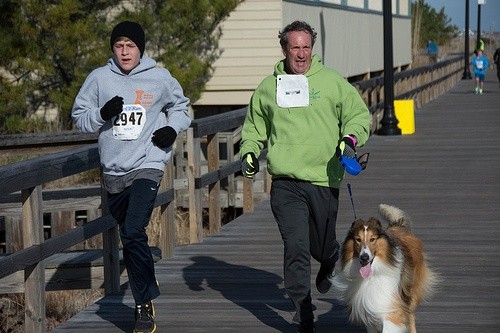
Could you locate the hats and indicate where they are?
[110,21,145,59]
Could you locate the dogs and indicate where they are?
[327,202,445,333]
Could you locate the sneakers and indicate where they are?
[316,265,335,294]
[133,300,156,333]
[298,322,316,333]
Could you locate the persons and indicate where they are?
[472,39,488,95]
[240,21,369,333]
[71,20,192,333]
[493,48,500,87]
[428,38,438,65]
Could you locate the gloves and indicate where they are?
[152,126,177,148]
[241,152,255,178]
[338,137,356,159]
[100,96,124,121]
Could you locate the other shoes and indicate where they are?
[479,89,482,93]
[475,87,479,92]
